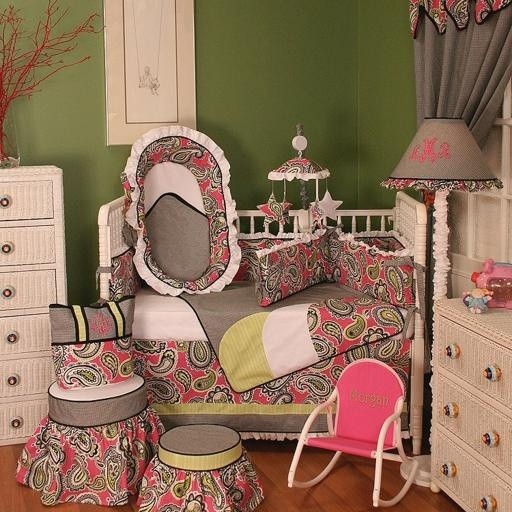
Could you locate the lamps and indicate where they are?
[379,116,504,488]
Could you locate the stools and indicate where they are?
[48,376,242,511]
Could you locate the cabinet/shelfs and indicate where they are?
[431,299,512,512]
[0,164,70,447]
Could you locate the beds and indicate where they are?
[95,189,432,455]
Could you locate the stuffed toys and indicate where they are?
[464,287,496,314]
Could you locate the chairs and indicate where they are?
[286,359,420,509]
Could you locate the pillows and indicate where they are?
[48,296,137,391]
[252,225,417,311]
